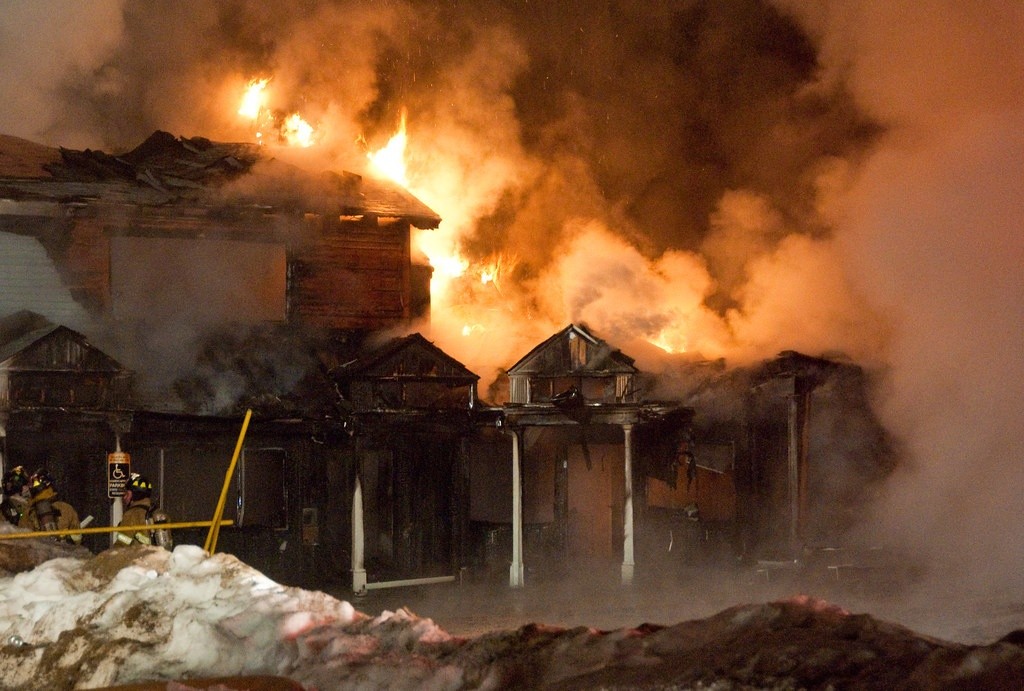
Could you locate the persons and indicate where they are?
[1,466,83,545]
[112,475,173,548]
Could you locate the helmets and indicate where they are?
[125,476,153,507]
[4,465,53,500]
